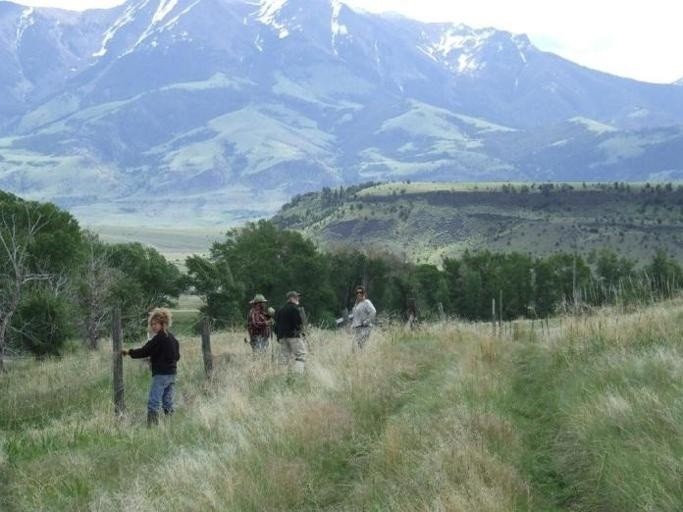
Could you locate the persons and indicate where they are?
[349,284,377,356]
[121,306,181,429]
[245,293,275,362]
[274,289,310,379]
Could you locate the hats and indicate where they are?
[248,292,269,305]
[286,291,302,298]
